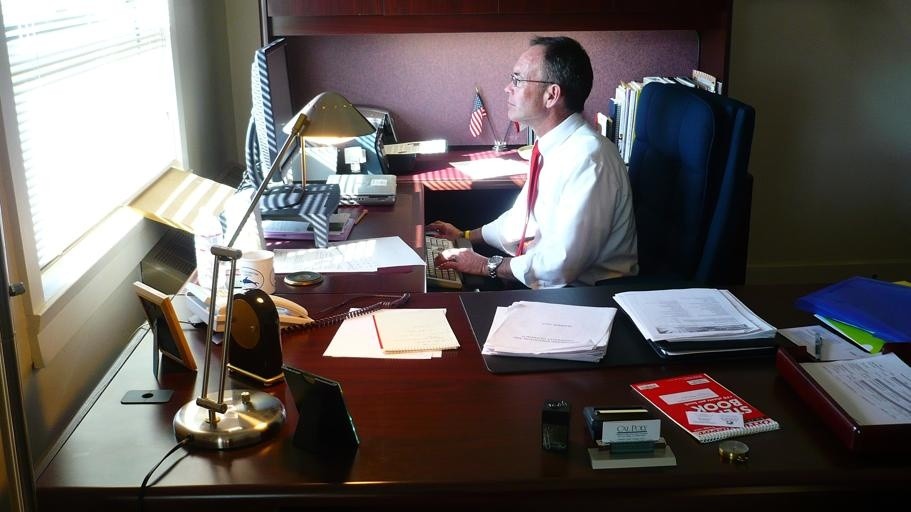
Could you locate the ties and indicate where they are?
[515,140,542,256]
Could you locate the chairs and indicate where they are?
[600,81,757,286]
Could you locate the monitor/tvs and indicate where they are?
[246,37,304,208]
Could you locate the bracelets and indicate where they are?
[463,229,470,242]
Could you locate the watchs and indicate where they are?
[487,253,504,279]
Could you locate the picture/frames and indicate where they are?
[278,360,363,484]
[131,280,198,371]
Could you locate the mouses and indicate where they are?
[425,229,440,236]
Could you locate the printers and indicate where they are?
[293,104,419,182]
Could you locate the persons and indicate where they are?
[425,35,641,291]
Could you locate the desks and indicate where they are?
[31,142,911,509]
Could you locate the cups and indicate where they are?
[237,249,277,297]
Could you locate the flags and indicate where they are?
[466,93,488,140]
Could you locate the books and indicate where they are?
[595,68,722,168]
[630,370,781,446]
[325,174,397,208]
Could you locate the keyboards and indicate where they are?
[425,236,464,289]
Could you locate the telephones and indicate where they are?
[185,282,315,333]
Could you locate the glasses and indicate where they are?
[509,74,557,87]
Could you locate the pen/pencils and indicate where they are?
[813,333,823,359]
[354,209,369,226]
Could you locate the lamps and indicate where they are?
[172,91,377,452]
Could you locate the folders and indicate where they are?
[794,275,911,345]
